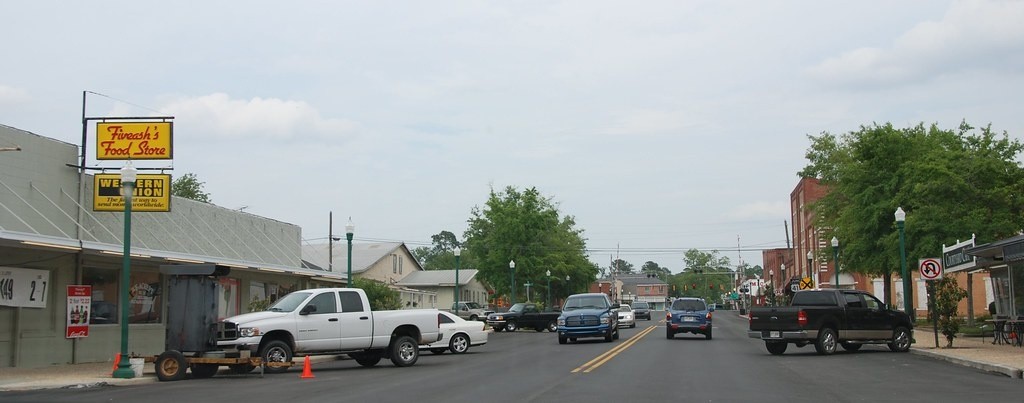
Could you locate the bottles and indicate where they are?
[71,304,88,325]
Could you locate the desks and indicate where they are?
[1006,319,1024,348]
[985,318,1011,347]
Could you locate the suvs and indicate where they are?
[557,292,621,344]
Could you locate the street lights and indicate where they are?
[344,214,354,288]
[743,281,747,315]
[566,275,571,296]
[509,259,516,305]
[629,291,631,306]
[806,250,813,289]
[546,269,551,312]
[831,236,840,289]
[894,207,916,344]
[454,246,460,316]
[769,269,774,307]
[112,157,136,379]
[621,289,624,303]
[748,278,752,308]
[781,263,786,307]
[599,283,602,292]
[757,274,760,306]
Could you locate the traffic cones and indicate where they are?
[111,353,122,374]
[298,355,316,379]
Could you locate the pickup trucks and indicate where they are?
[215,286,445,375]
[746,287,914,357]
[486,301,562,333]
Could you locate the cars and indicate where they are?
[665,296,715,340]
[632,301,653,321]
[449,301,495,324]
[418,309,489,355]
[617,303,636,328]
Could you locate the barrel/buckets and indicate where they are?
[129,358,145,377]
